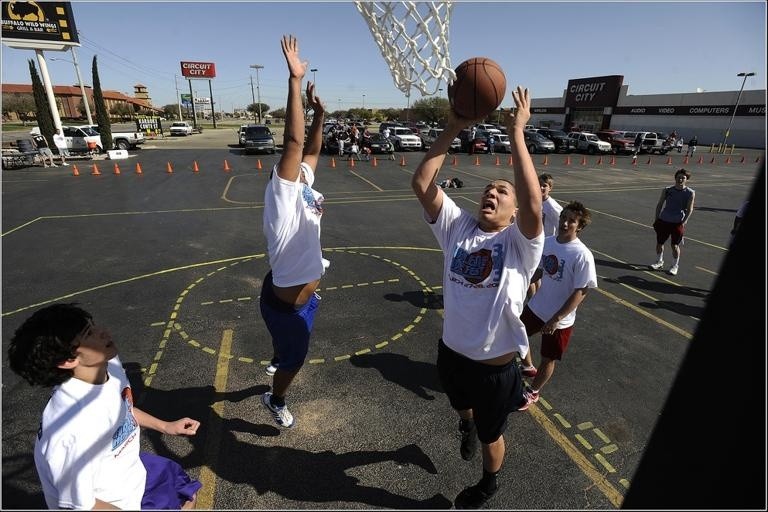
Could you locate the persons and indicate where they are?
[515,201,599,412]
[32,129,57,168]
[383,126,391,138]
[527,174,565,303]
[9,302,201,511]
[467,127,476,155]
[488,132,495,156]
[649,169,695,276]
[386,139,396,160]
[731,198,750,235]
[411,85,546,509]
[687,136,697,157]
[634,134,642,156]
[53,129,70,166]
[332,124,374,160]
[668,130,684,154]
[260,32,325,428]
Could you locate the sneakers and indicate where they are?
[458,419,477,462]
[517,386,539,411]
[521,365,538,377]
[454,479,501,510]
[647,261,665,270]
[260,392,295,429]
[264,362,278,376]
[668,264,680,275]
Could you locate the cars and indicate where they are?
[167,118,197,135]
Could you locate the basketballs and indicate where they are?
[448,57,506,120]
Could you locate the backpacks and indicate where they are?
[451,178,463,188]
[440,177,451,188]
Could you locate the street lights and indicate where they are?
[310,68,318,97]
[437,88,444,119]
[718,71,756,153]
[362,95,366,109]
[249,64,264,124]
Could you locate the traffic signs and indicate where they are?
[49,57,93,126]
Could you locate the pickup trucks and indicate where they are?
[30,125,147,151]
